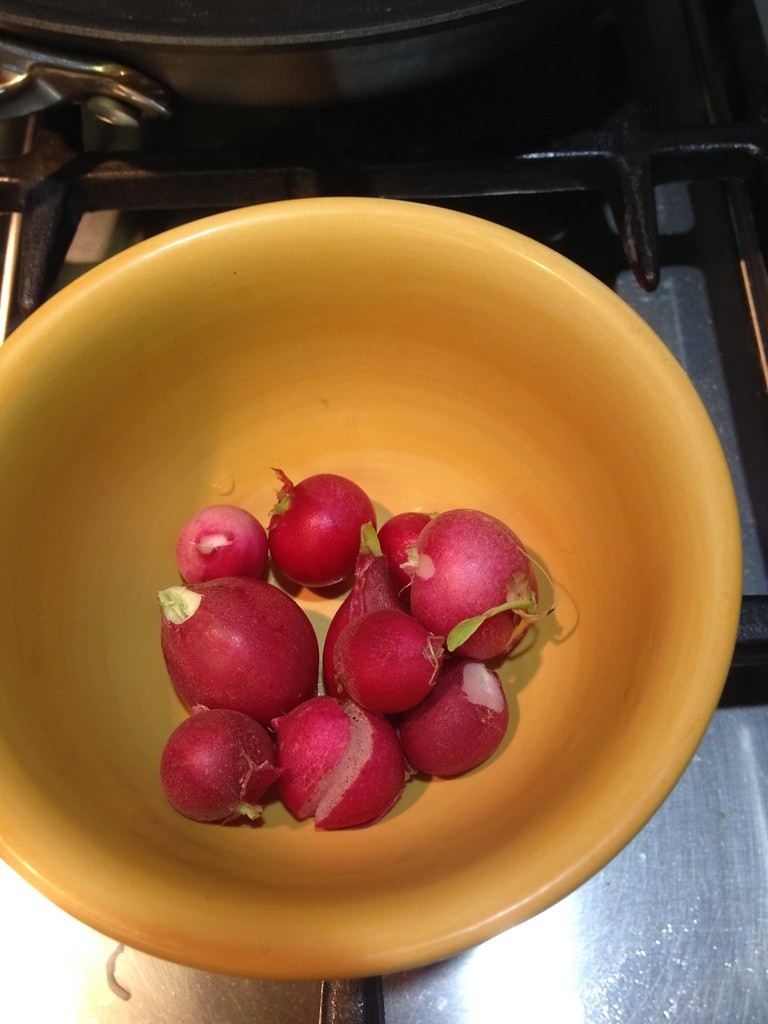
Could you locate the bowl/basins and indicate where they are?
[0,191,742,980]
